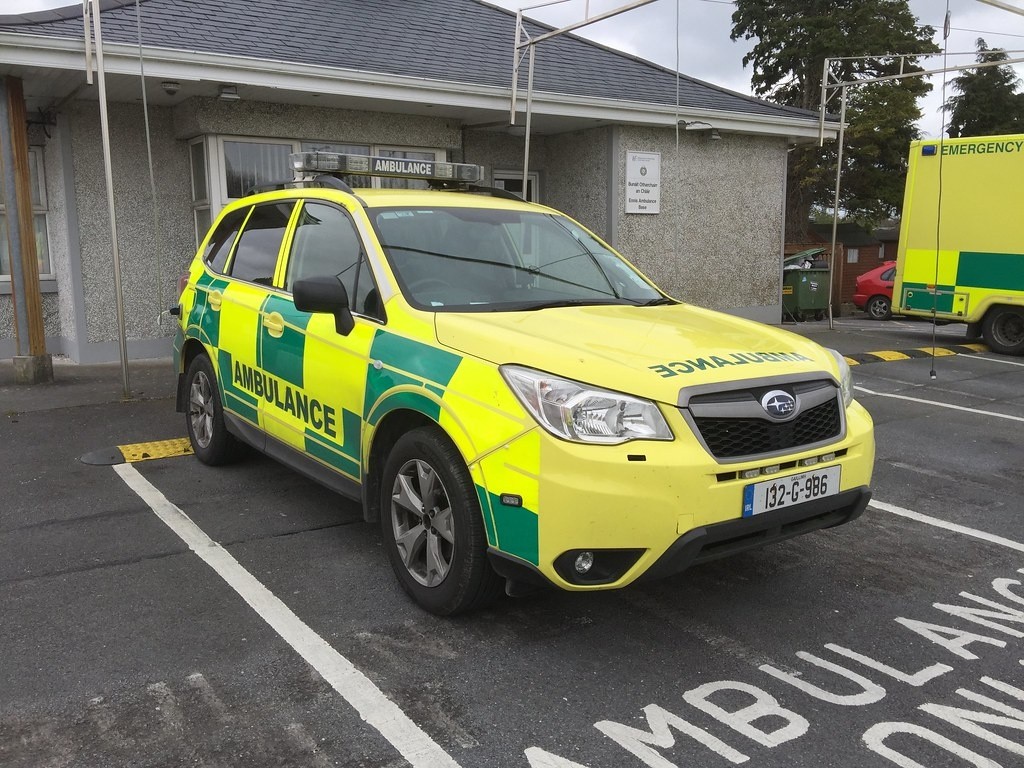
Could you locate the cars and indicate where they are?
[852,260,897,323]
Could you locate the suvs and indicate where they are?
[169,152,878,621]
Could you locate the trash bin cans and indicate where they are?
[782,248,829,321]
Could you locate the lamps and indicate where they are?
[217,85,241,105]
[708,129,721,139]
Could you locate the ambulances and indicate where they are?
[891,131,1024,355]
